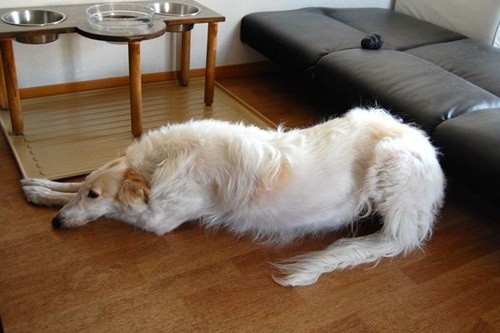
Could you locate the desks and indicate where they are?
[1,0,225,140]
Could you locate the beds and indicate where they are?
[243,0,499,213]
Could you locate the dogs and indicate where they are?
[19,105,448,289]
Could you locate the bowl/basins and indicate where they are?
[85,2,156,46]
[149,2,201,32]
[1,9,67,45]
